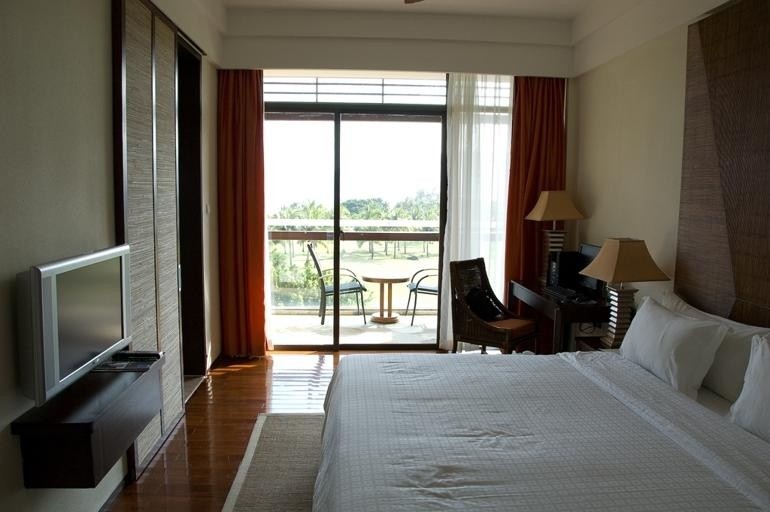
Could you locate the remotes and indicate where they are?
[112,351,163,359]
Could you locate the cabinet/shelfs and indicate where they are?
[9,350,166,487]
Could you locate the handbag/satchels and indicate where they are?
[465,286,505,320]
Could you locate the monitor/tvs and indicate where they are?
[574,242,603,297]
[16,243,134,408]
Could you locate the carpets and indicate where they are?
[221,410,325,511]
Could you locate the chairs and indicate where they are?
[307,241,367,325]
[450,258,541,354]
[403,269,440,326]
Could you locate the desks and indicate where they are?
[506,277,612,353]
[362,275,413,324]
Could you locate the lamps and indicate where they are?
[523,190,584,287]
[579,237,671,349]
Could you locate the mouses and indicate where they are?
[576,296,584,302]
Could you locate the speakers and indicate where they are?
[549,251,577,287]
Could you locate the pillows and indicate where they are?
[617,290,769,445]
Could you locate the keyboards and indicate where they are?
[543,287,577,301]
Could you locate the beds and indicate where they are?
[310,351,770,510]
